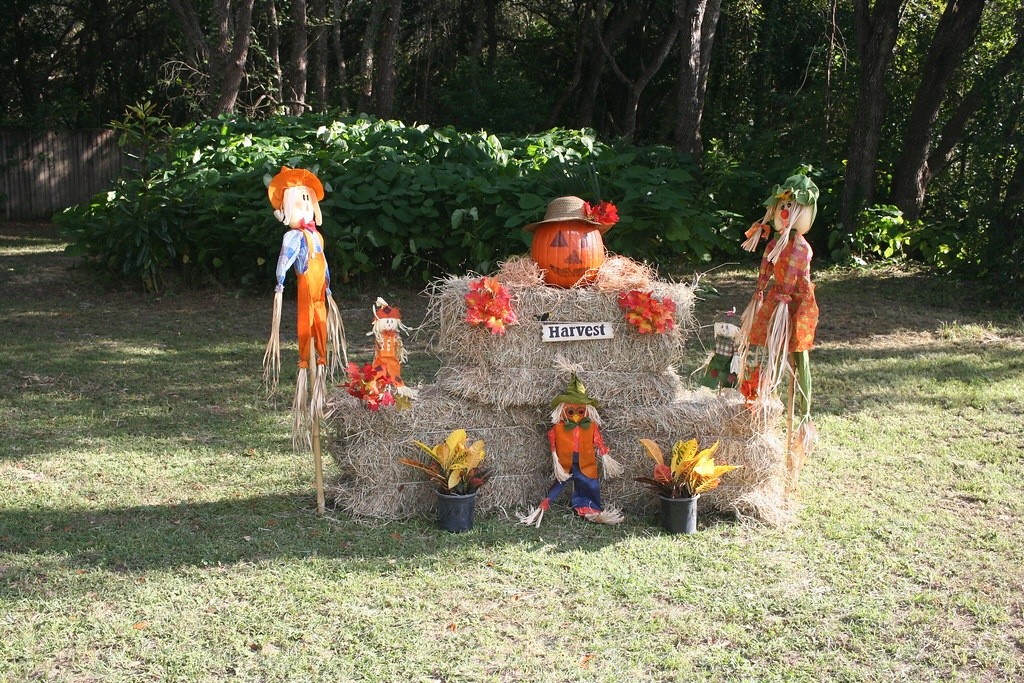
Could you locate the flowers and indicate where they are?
[585,199,620,226]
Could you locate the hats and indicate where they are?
[523,195,615,234]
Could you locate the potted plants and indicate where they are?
[399,429,498,534]
[633,438,743,534]
